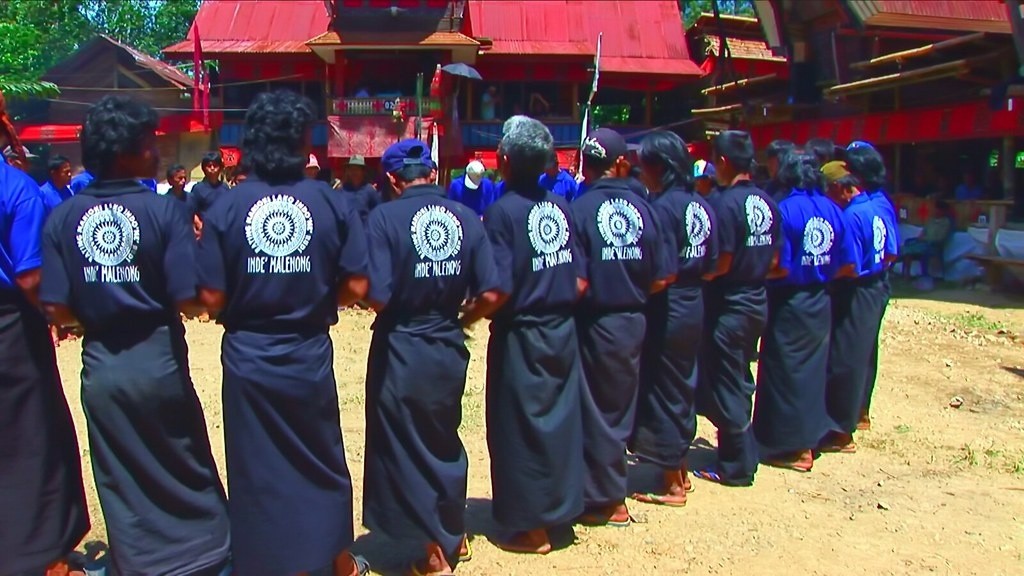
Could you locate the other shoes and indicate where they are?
[344,550,372,576]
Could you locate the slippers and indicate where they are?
[576,506,632,526]
[854,420,872,428]
[817,438,857,453]
[452,533,471,561]
[407,550,454,576]
[692,464,744,485]
[629,474,695,507]
[761,455,808,473]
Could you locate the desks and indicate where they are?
[938,198,1015,232]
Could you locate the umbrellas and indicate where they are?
[441,62,483,97]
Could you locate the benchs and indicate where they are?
[964,254,1024,291]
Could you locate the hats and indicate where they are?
[464,161,485,189]
[583,127,631,166]
[692,159,715,180]
[306,154,321,171]
[819,160,854,183]
[379,139,434,184]
[847,140,874,153]
[345,153,367,169]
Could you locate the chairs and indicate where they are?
[902,236,945,282]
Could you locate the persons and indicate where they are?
[819,161,888,455]
[905,200,951,279]
[954,170,984,200]
[459,115,586,555]
[527,91,551,118]
[845,140,901,429]
[630,130,719,507]
[693,130,782,486]
[0,85,91,576]
[41,94,231,576]
[752,149,858,474]
[572,128,670,526]
[362,138,501,576]
[196,86,372,576]
[0,140,850,348]
[481,85,502,120]
